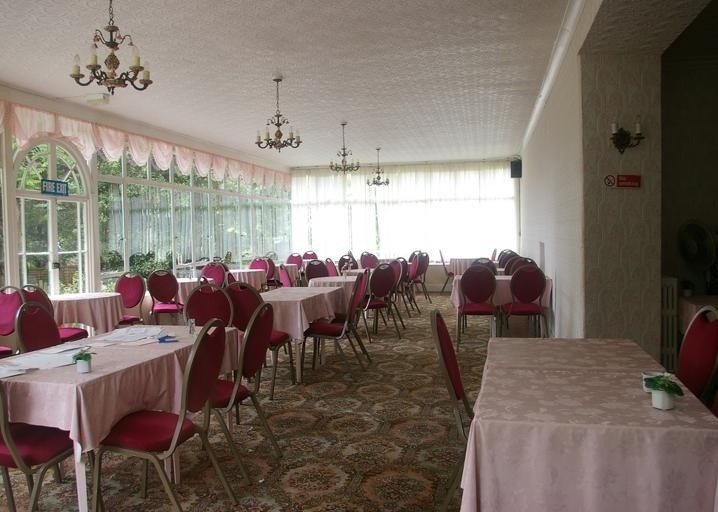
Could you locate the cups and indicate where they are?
[188,319,196,335]
[640,367,671,393]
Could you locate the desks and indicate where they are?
[47,291,126,338]
[3,322,242,510]
[460,327,718,511]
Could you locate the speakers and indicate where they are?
[510,160,522,178]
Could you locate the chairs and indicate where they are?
[149,268,184,325]
[187,300,284,482]
[428,310,478,437]
[111,271,148,325]
[436,243,557,342]
[675,305,718,403]
[2,282,88,510]
[178,248,431,401]
[90,318,251,511]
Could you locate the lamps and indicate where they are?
[65,0,156,100]
[609,116,646,154]
[255,72,304,154]
[366,144,391,188]
[329,119,363,174]
